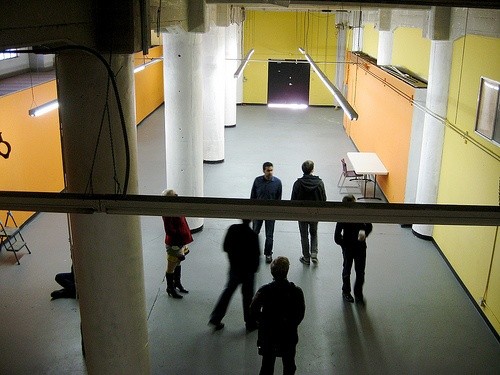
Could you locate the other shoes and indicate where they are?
[342,292,355,303]
[266,255,272,263]
[209,316,224,330]
[311,256,319,263]
[245,321,258,332]
[300,255,310,265]
[353,293,364,304]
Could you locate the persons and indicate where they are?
[334,194,372,303]
[209,219,259,332]
[50,254,76,298]
[251,162,282,263]
[248,255,306,375]
[291,161,326,266]
[161,188,193,299]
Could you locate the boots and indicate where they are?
[165,272,184,298]
[174,266,188,293]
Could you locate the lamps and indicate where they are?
[134,55,163,74]
[28,47,59,118]
[298,0,362,121]
[233,0,255,79]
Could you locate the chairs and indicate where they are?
[337,158,364,196]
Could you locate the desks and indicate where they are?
[347,152,389,200]
